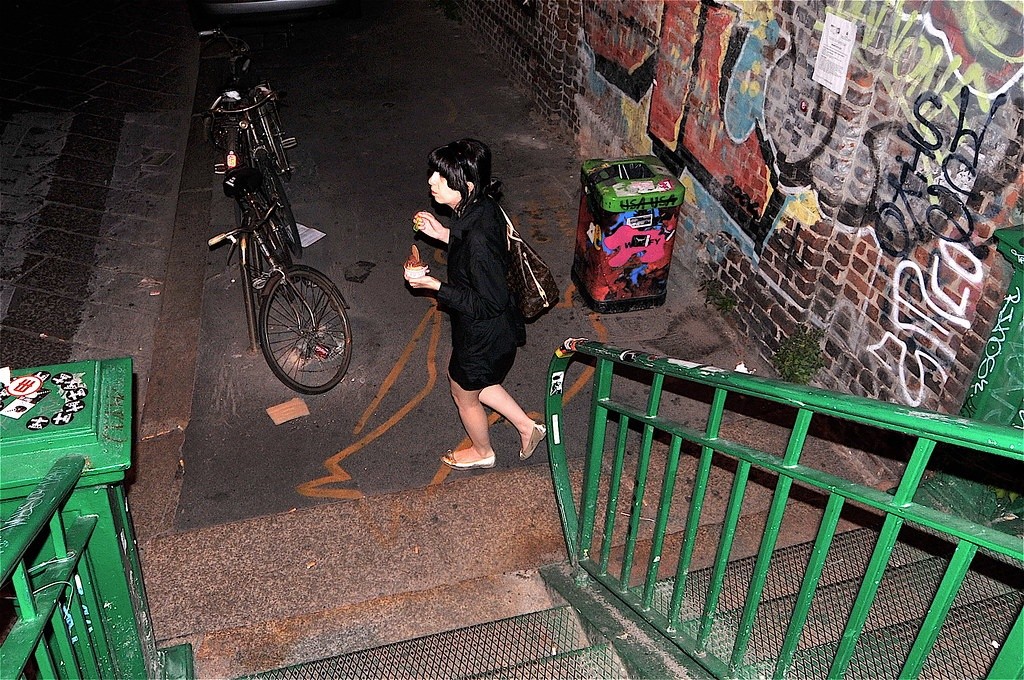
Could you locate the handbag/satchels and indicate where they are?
[489,194,560,318]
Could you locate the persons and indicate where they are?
[403,138,548,471]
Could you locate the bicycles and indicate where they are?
[207,147,352,395]
[200,78,303,258]
[196,29,253,143]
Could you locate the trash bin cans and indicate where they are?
[570,156,686,315]
[0,356,196,680]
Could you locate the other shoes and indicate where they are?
[519,421,547,460]
[440,449,496,469]
